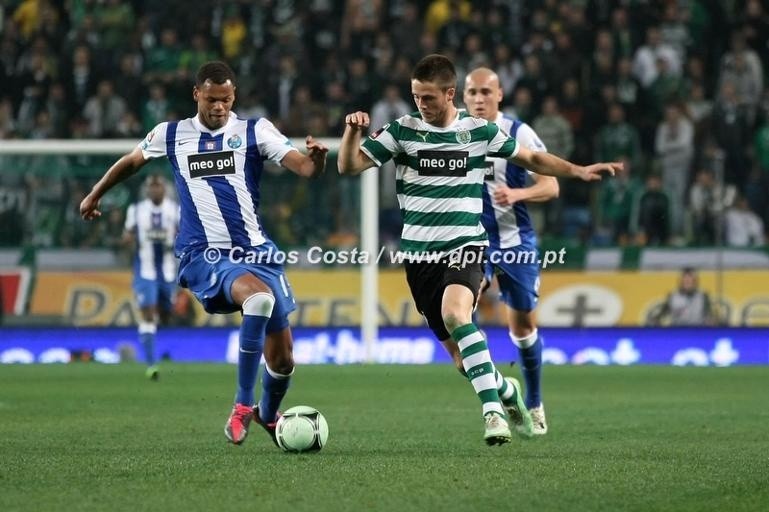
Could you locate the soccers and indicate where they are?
[274,405,330,454]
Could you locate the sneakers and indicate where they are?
[145,365,160,382]
[500,374,536,441]
[253,403,287,448]
[481,412,514,448]
[222,401,255,444]
[522,398,550,437]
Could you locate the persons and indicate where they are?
[2,0,768,273]
[335,52,626,446]
[648,268,718,327]
[463,65,560,436]
[118,172,181,384]
[80,59,328,451]
